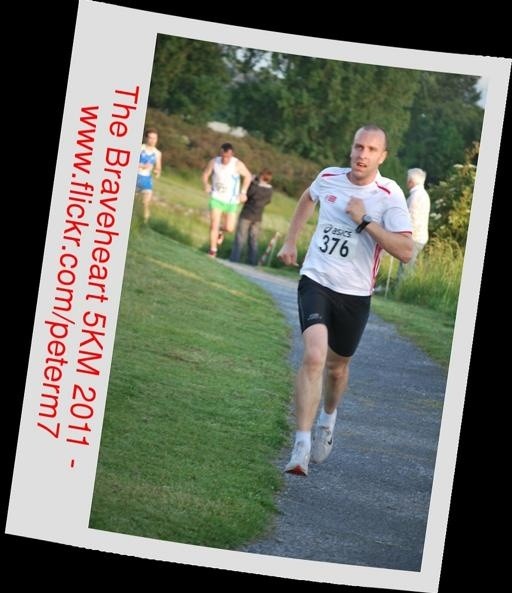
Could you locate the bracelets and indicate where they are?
[241,188,247,193]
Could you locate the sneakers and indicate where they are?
[209,248,218,258]
[216,230,224,247]
[284,440,312,477]
[311,419,336,465]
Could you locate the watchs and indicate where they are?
[356,214,372,233]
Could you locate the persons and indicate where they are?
[392,166,431,303]
[277,122,415,474]
[228,165,274,265]
[134,127,163,223]
[201,141,252,257]
